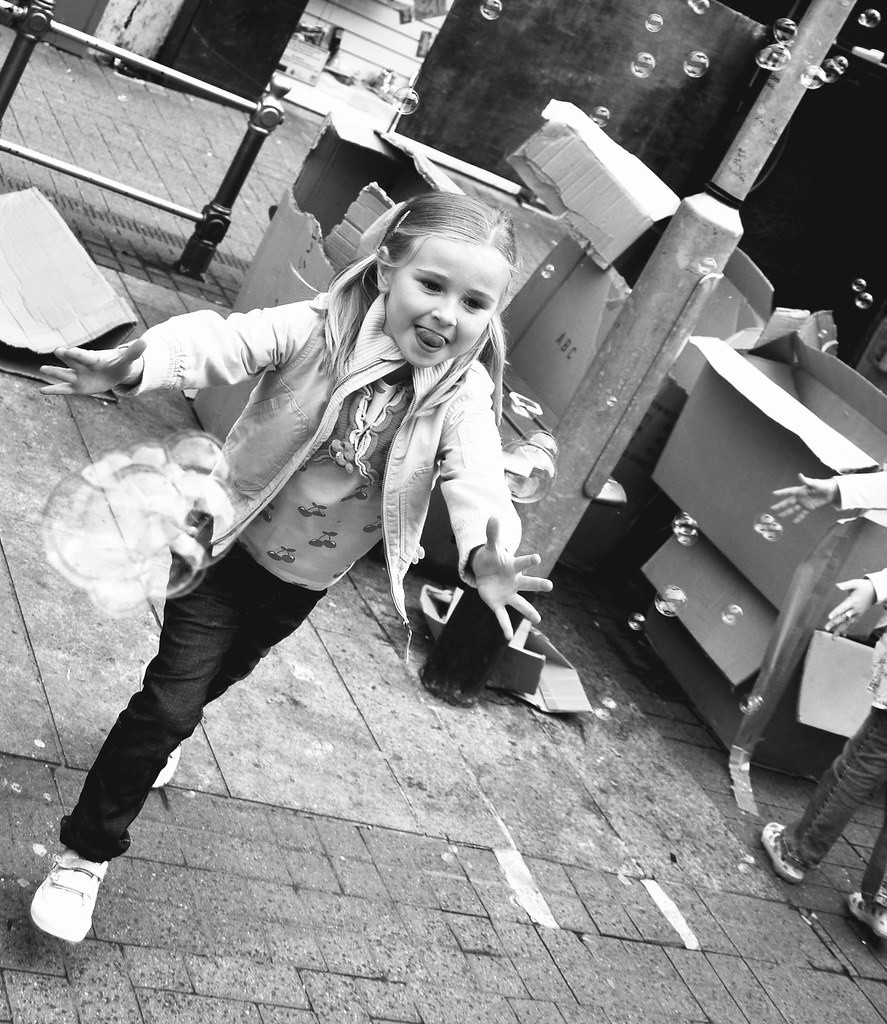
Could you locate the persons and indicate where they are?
[763,473,887,937]
[31,192,553,942]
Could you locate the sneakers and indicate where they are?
[152,740,181,788]
[762,822,804,883]
[31,846,108,941]
[848,892,887,938]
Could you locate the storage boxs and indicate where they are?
[642,530,875,777]
[651,336,887,637]
[191,96,777,571]
